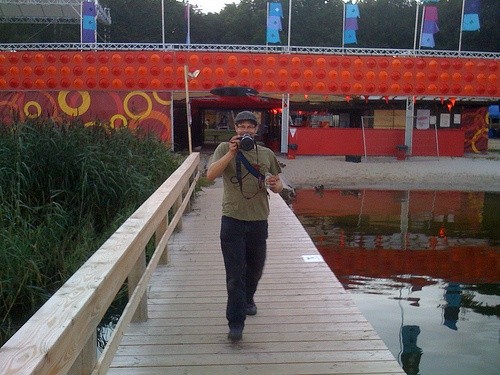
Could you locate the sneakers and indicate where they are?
[228,326,243,340]
[246,301,258,315]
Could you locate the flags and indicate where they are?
[82,1,96,16]
[267,30,281,43]
[269,2,284,18]
[268,16,283,31]
[462,14,481,32]
[83,17,97,30]
[345,18,359,30]
[424,20,440,33]
[82,30,96,43]
[346,4,360,18]
[183,6,188,33]
[425,7,438,20]
[464,0,480,14]
[421,33,436,48]
[344,30,357,44]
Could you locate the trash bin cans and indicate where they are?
[397,144,408,160]
[269,137,280,152]
[288,144,297,159]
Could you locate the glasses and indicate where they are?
[237,123,256,131]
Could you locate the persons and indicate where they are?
[206,111,283,342]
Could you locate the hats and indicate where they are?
[235,110,257,126]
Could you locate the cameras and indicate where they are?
[237,134,256,151]
